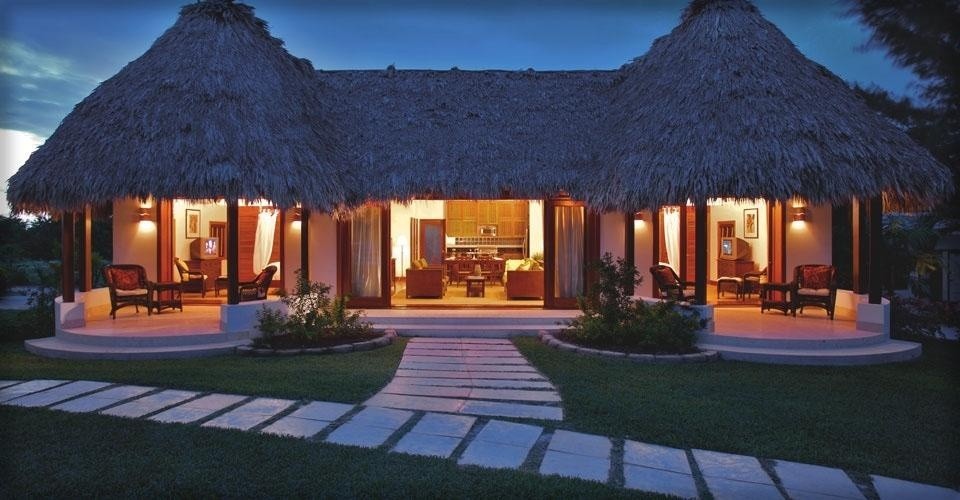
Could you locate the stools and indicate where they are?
[717,277,743,300]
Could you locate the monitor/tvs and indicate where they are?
[190,237,219,260]
[720,237,750,260]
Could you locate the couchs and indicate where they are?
[503,257,544,301]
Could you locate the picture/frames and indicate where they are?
[743,208,758,239]
[185,209,201,239]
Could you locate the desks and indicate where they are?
[445,257,503,283]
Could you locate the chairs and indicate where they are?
[791,264,838,320]
[649,265,696,302]
[449,253,497,288]
[743,266,768,299]
[101,264,153,320]
[173,256,207,299]
[406,264,448,299]
[238,265,277,302]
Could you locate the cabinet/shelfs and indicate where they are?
[717,259,755,294]
[183,260,222,291]
[419,219,446,266]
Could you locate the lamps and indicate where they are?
[635,214,643,221]
[793,207,806,222]
[138,207,151,221]
[288,208,301,223]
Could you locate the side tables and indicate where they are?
[215,278,229,297]
[761,283,795,316]
[147,281,183,316]
